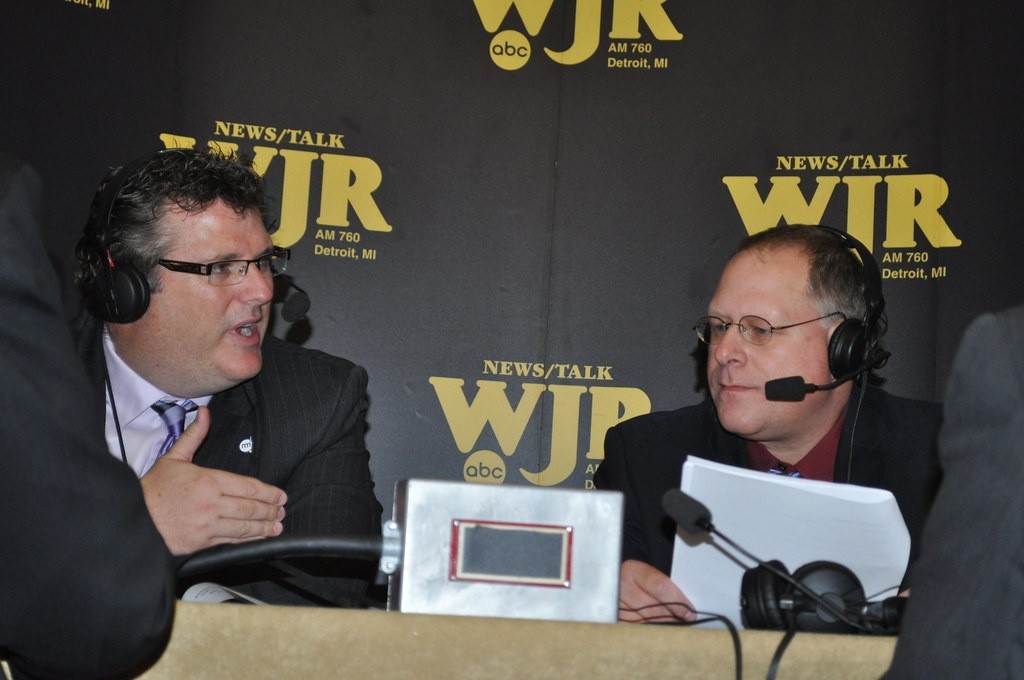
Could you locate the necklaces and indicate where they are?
[101,334,127,464]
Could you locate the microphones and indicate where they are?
[662,489,893,636]
[273,281,311,322]
[765,350,891,403]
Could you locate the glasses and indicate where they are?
[692,312,846,346]
[155,245,291,287]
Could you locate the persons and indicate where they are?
[0,156,176,680]
[593,223,943,637]
[877,305,1024,680]
[69,140,384,609]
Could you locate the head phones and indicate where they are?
[741,559,909,637]
[814,223,892,382]
[77,147,177,324]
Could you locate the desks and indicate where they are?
[136,600,897,680]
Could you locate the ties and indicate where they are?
[770,464,800,478]
[150,398,199,462]
